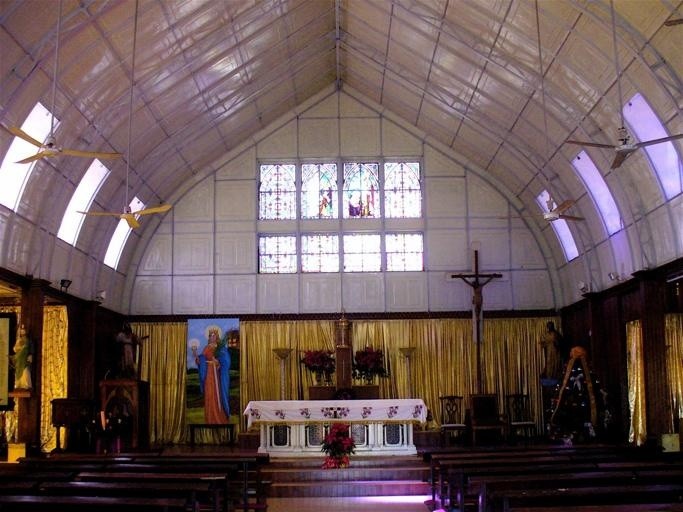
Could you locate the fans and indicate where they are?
[9,0,124,164]
[498,0,584,221]
[565,0,683,169]
[76,0,171,228]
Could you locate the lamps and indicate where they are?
[96,290,106,302]
[607,272,626,282]
[61,279,72,293]
[577,280,588,293]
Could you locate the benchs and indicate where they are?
[421,444,683,512]
[0,452,271,512]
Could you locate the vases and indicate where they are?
[315,371,333,386]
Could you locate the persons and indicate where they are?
[11,322,32,395]
[104,386,133,455]
[191,326,234,426]
[458,273,497,323]
[112,321,147,380]
[539,320,565,383]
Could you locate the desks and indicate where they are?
[243,399,427,455]
[189,423,237,453]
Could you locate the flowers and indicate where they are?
[301,350,335,374]
[352,344,390,380]
[320,423,356,470]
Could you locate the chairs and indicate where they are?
[507,394,537,441]
[439,396,467,446]
[469,394,507,446]
